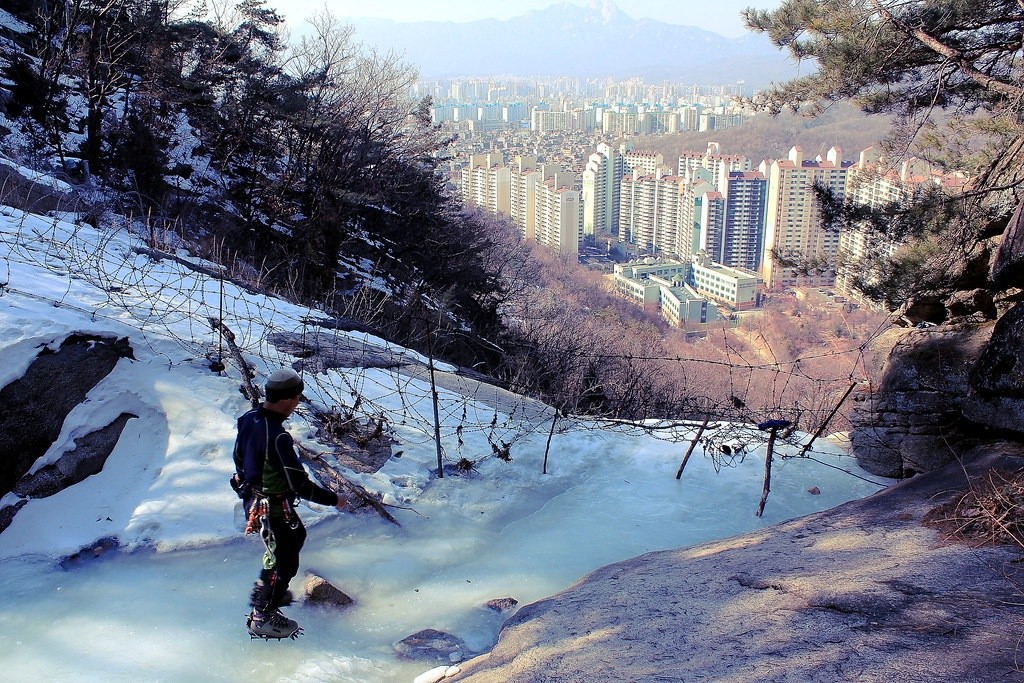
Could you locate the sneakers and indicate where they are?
[248,582,293,608]
[245,607,304,642]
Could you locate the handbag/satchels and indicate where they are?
[262,459,293,494]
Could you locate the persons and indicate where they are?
[230,368,349,642]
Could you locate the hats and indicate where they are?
[265,367,304,400]
